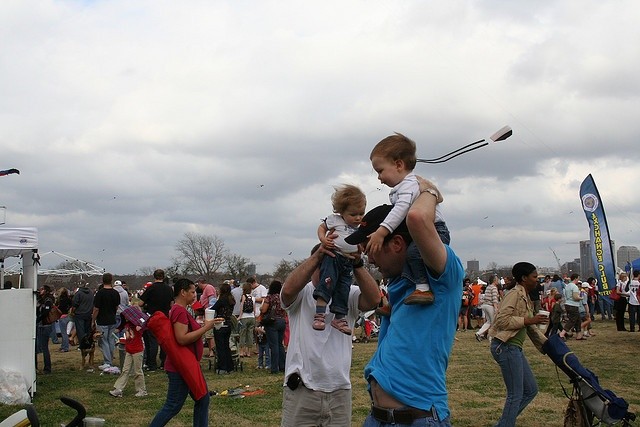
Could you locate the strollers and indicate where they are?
[541,333,636,426]
[208,315,243,371]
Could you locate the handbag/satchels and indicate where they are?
[145,311,208,401]
[564,399,593,427]
[260,308,276,324]
[43,305,62,324]
[610,287,622,301]
[464,295,469,306]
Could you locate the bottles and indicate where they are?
[205,310,215,321]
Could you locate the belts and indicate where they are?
[371,404,432,424]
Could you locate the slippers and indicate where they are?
[576,336,586,340]
[558,333,566,341]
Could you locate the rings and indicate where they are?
[213,323,216,326]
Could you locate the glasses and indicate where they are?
[39,288,45,291]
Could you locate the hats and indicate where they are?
[195,276,206,282]
[114,280,122,285]
[76,280,85,288]
[582,282,593,289]
[344,205,408,245]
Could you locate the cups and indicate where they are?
[538,310,551,330]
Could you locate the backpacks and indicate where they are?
[241,294,254,313]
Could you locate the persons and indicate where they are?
[121,280,132,307]
[580,281,597,337]
[244,277,267,320]
[260,279,287,374]
[35,284,61,375]
[490,261,552,427]
[475,272,500,344]
[210,282,236,374]
[72,278,95,372]
[615,271,629,333]
[3,280,13,290]
[56,287,72,352]
[466,276,475,331]
[545,292,574,342]
[626,268,640,331]
[92,272,121,370]
[538,275,552,294]
[312,182,366,336]
[254,318,272,370]
[366,131,441,306]
[279,244,383,427]
[458,279,468,333]
[112,279,129,345]
[109,308,149,398]
[346,204,466,426]
[236,283,257,358]
[140,268,175,372]
[473,285,487,330]
[548,273,567,301]
[230,279,243,342]
[151,277,226,426]
[588,278,597,320]
[558,273,586,341]
[196,277,217,358]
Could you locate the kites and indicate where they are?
[415,122,513,166]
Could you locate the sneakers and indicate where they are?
[332,318,351,335]
[583,333,590,337]
[109,388,122,397]
[312,314,326,331]
[68,338,75,346]
[135,390,148,397]
[376,305,390,315]
[99,364,110,370]
[403,289,434,305]
[475,333,481,342]
